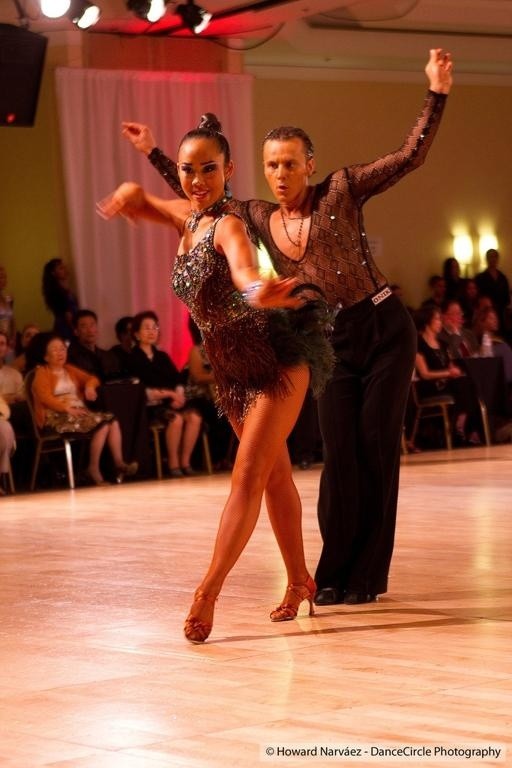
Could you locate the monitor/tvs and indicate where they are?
[0,23,48,129]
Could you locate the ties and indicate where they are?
[460,342,470,360]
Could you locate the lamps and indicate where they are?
[176,0,212,35]
[126,0,167,23]
[480,236,499,258]
[67,0,101,30]
[40,0,70,18]
[453,235,474,264]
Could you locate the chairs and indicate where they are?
[409,383,491,452]
[3,457,15,494]
[23,369,92,491]
[148,422,213,480]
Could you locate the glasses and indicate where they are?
[47,347,68,352]
[138,326,160,334]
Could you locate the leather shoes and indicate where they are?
[316,585,345,605]
[344,585,376,602]
[455,425,466,437]
[86,469,109,486]
[470,438,484,445]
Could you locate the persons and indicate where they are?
[383,244,512,454]
[121,44,454,607]
[92,111,338,644]
[1,251,222,495]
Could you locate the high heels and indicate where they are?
[184,589,219,643]
[270,575,317,621]
[117,461,138,477]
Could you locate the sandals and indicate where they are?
[182,466,201,476]
[166,467,185,477]
